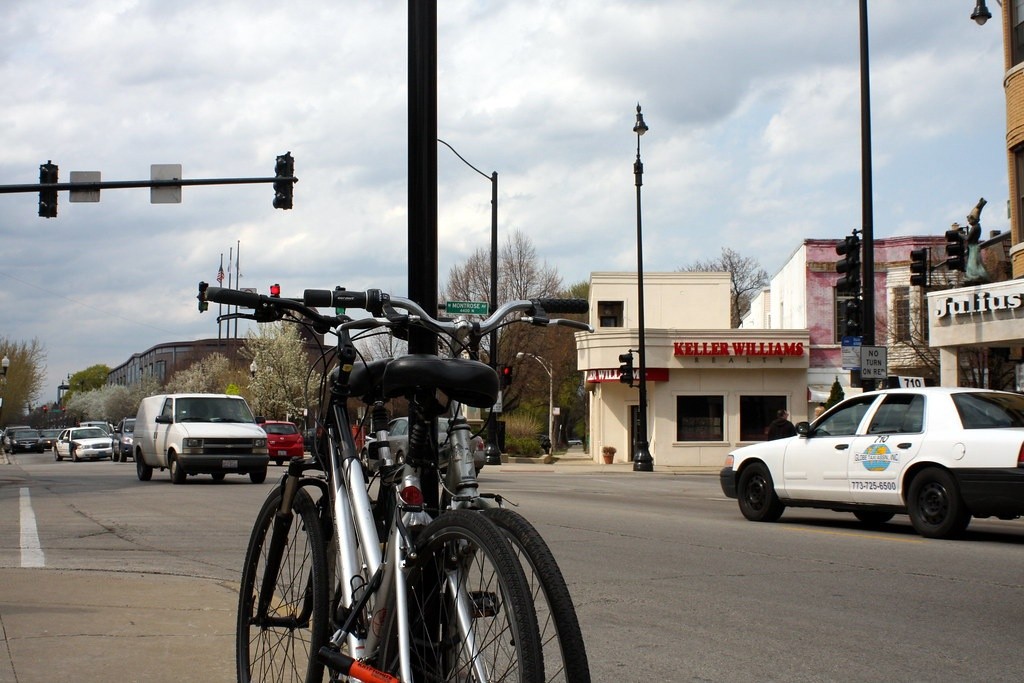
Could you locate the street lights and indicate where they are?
[516,351,553,454]
[633,101,653,471]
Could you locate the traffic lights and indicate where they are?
[618,352,633,387]
[909,247,927,287]
[196,281,211,313]
[270,284,281,299]
[946,228,966,272]
[501,366,511,386]
[835,235,861,294]
[335,285,345,316]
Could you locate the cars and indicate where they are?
[9,428,45,454]
[257,420,304,466]
[40,428,63,449]
[360,415,486,479]
[51,426,114,463]
[720,386,1024,539]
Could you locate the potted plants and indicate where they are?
[602,446,616,464]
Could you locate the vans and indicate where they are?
[78,421,114,438]
[132,393,270,485]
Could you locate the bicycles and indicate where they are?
[202,286,594,683]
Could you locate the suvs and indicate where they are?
[4,426,32,440]
[111,417,136,462]
